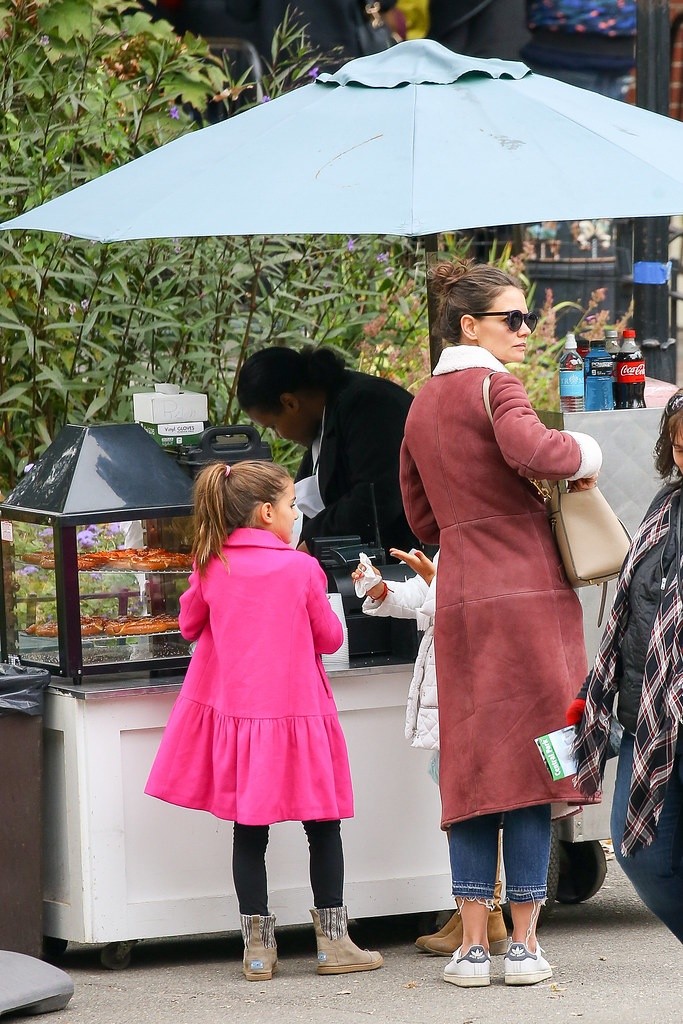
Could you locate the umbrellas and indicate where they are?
[0,39,682,371]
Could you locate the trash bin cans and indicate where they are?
[0,663,63,956]
[526,261,617,339]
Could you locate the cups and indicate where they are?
[322,593,349,666]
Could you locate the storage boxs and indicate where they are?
[137,422,205,447]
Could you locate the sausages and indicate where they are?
[20,549,196,571]
[25,616,181,637]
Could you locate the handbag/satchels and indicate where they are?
[482,371,635,588]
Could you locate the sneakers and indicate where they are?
[504,938,553,984]
[442,943,491,987]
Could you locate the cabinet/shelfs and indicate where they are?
[0,505,200,677]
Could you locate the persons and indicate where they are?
[144,460,382,979]
[237,347,420,554]
[567,389,683,945]
[352,548,509,955]
[399,264,602,988]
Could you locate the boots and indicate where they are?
[415,827,508,956]
[238,910,278,980]
[309,904,382,975]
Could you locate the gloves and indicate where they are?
[564,697,586,727]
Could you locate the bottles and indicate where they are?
[559,333,585,413]
[616,329,647,410]
[604,330,620,410]
[584,339,614,412]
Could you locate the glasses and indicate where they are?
[454,309,540,336]
[662,394,683,429]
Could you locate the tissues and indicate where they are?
[133,383,208,424]
